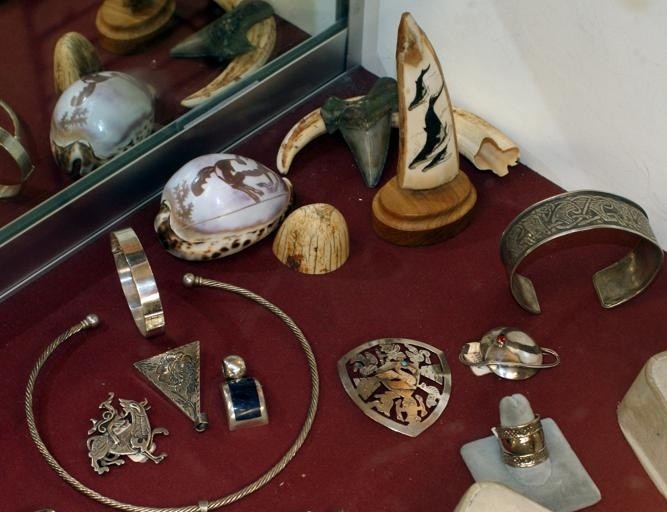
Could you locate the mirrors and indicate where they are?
[0,0,364,298]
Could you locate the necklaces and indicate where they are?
[25,273,320,512]
[0,98,22,142]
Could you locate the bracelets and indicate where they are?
[109,227,167,339]
[500,188,664,315]
[0,128,36,199]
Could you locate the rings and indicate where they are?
[494,413,548,468]
